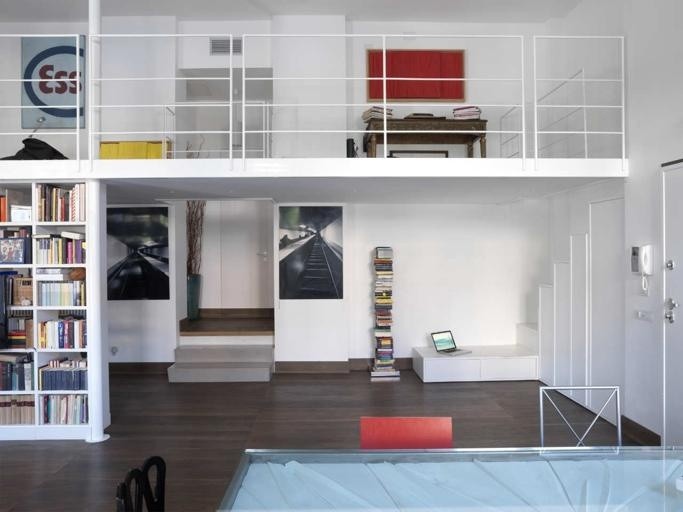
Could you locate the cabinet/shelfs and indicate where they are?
[0,180,112,443]
[411,346,540,382]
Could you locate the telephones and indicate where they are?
[630,245,652,276]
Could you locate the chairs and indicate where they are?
[539,385,621,447]
[360,416,452,448]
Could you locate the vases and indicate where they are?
[188,274,201,321]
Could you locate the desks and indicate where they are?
[214,446,682,512]
[363,119,488,158]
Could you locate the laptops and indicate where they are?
[430,330,472,357]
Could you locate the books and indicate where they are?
[451,106,480,120]
[361,106,393,123]
[367,245,400,381]
[0,181,88,427]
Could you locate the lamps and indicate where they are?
[233,89,242,148]
